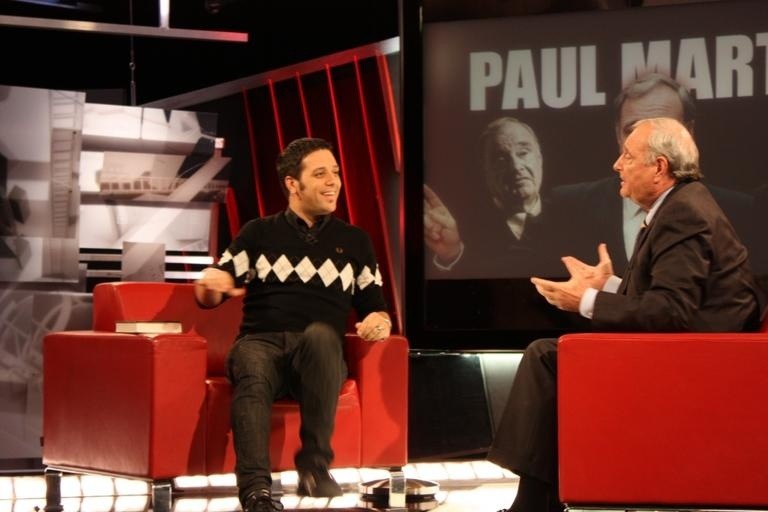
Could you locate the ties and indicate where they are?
[639,219,647,233]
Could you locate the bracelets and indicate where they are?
[379,314,392,328]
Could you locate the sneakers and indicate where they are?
[241,489,278,512]
[296,469,344,498]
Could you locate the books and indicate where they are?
[114,320,182,335]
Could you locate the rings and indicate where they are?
[375,325,383,332]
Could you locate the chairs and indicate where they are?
[42,281,410,512]
[550,311,767,509]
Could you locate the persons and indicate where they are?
[488,118,759,512]
[424,70,733,278]
[471,112,546,256]
[197,135,393,512]
